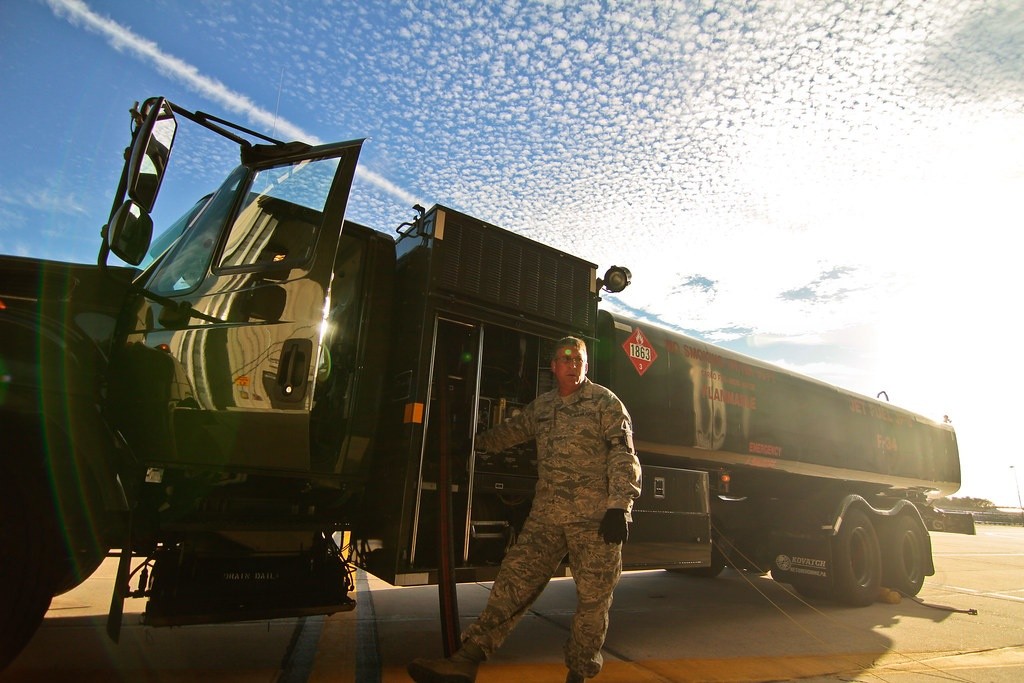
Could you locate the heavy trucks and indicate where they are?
[97,97,975,662]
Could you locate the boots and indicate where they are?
[408,636,483,683]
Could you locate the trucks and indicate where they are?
[0,255,134,673]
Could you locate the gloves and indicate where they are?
[598,509,629,545]
[453,439,473,473]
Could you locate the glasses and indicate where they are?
[554,356,587,365]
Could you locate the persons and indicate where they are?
[404,336,643,683]
